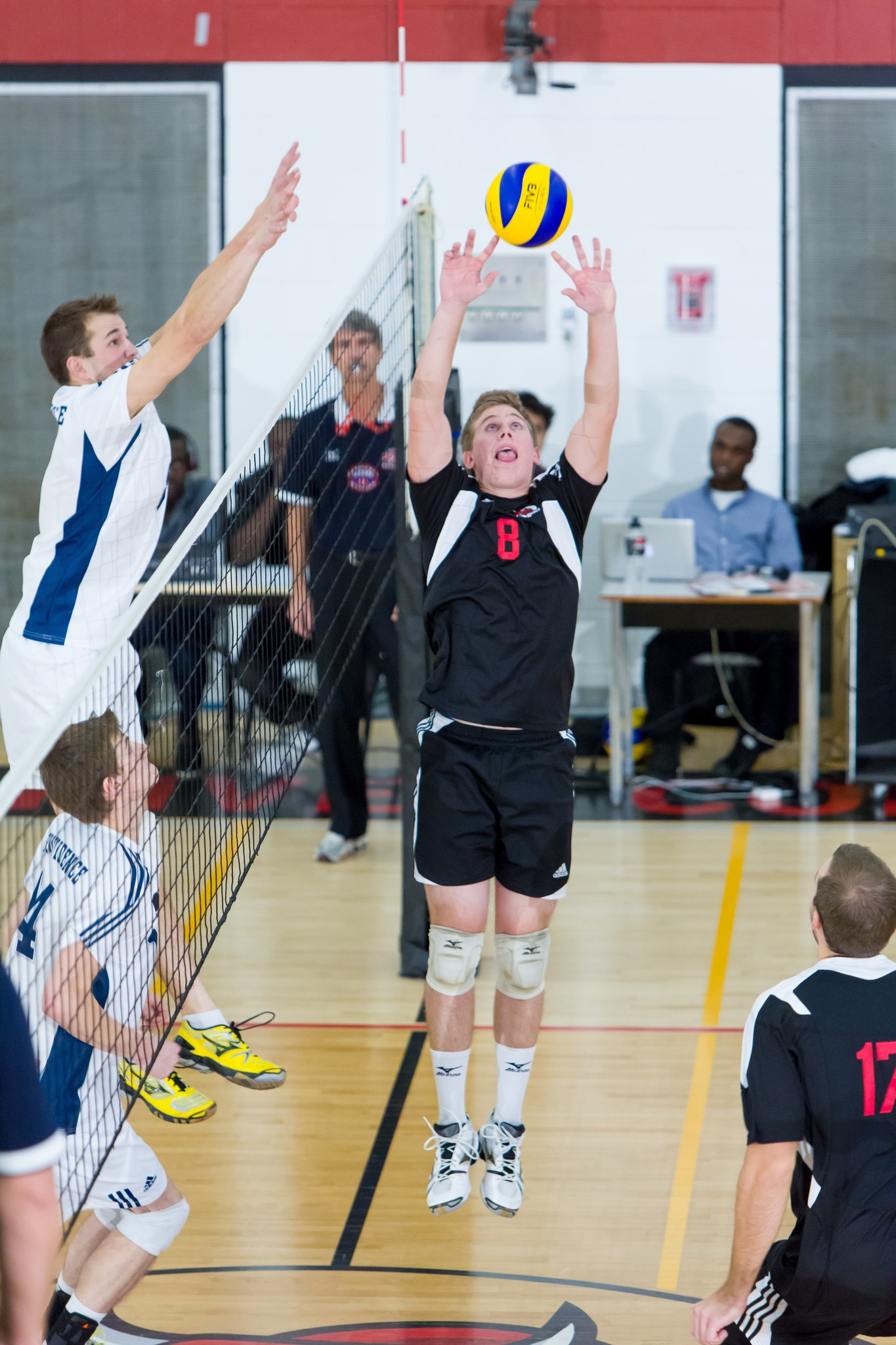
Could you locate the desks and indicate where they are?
[599,570,830,808]
[133,583,291,765]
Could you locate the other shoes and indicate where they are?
[175,709,203,778]
[634,754,681,781]
[713,746,759,775]
[316,832,367,861]
[254,726,313,777]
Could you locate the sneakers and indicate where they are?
[117,1056,216,1125]
[173,1012,286,1088]
[478,1109,525,1218]
[423,1107,481,1214]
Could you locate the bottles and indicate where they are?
[624,516,649,595]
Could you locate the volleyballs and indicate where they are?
[485,162,572,247]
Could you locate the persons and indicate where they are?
[403,228,620,1218]
[0,141,303,1124]
[126,418,322,780]
[0,719,193,1345]
[687,844,896,1345]
[632,416,807,779]
[273,310,398,863]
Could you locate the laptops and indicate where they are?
[599,517,700,580]
